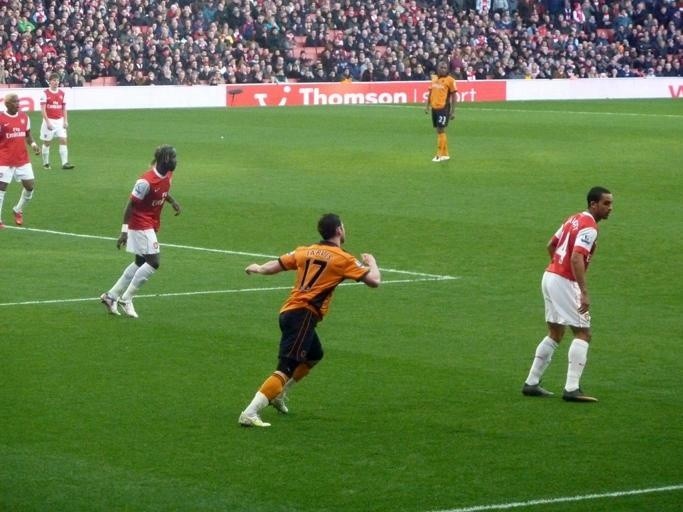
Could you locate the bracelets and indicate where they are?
[121,223,129,233]
[31,142,37,148]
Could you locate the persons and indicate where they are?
[38,73,76,171]
[238,212,382,434]
[0,92,42,229]
[522,186,613,401]
[100,144,182,319]
[424,61,458,162]
[1,1,683,88]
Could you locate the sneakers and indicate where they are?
[522,382,554,396]
[117,296,138,318]
[432,155,449,161]
[562,387,597,403]
[238,411,271,427]
[62,163,75,169]
[269,397,288,414]
[43,164,51,170]
[100,292,121,316]
[16,209,23,225]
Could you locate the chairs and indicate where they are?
[596,28,615,43]
[83,76,117,86]
[0,83,25,87]
[293,36,325,58]
[375,46,388,55]
